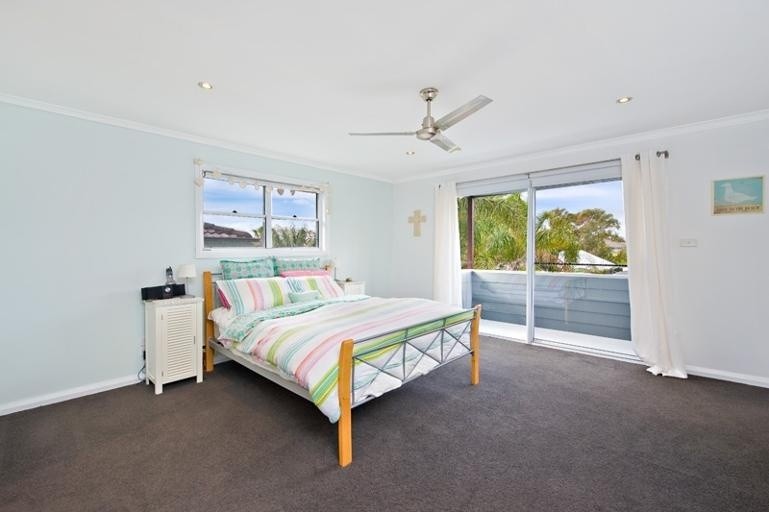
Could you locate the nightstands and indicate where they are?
[338,280,365,297]
[143,294,204,395]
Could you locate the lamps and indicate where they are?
[178,264,198,299]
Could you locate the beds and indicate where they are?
[204,270,482,467]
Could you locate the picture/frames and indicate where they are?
[711,175,765,216]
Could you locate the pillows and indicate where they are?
[215,256,344,315]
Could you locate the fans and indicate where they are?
[348,88,494,154]
